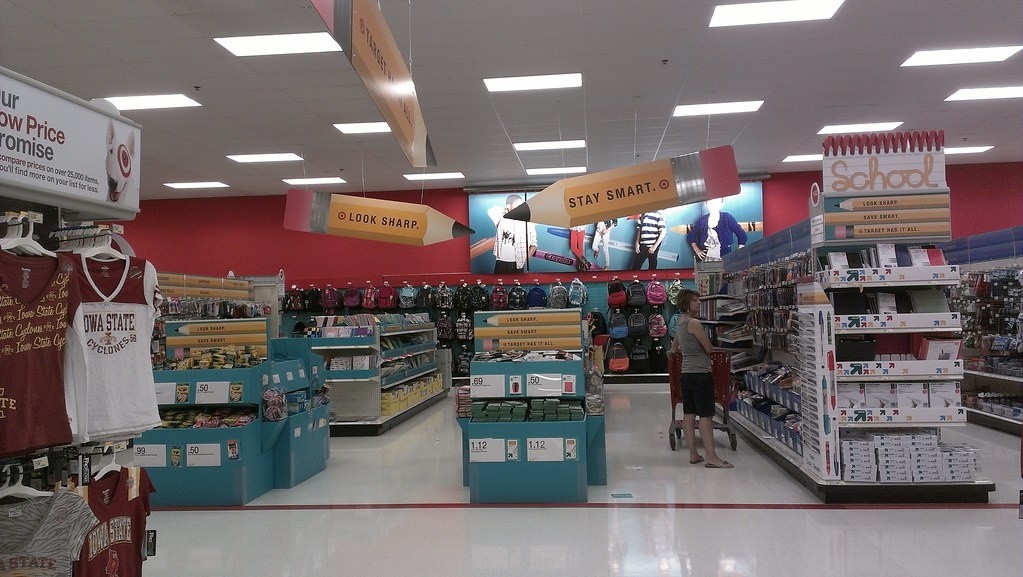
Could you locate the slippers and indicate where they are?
[690,453,704,464]
[705,459,735,468]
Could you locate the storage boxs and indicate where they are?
[824,244,981,483]
[382,374,444,415]
[472,397,584,422]
[734,397,803,455]
[744,373,803,415]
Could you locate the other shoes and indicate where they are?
[603,266,608,270]
[594,247,600,259]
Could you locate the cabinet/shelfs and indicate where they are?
[270,336,331,459]
[311,321,453,436]
[939,226,1022,436]
[469,310,588,501]
[715,218,997,505]
[270,357,328,488]
[129,358,274,507]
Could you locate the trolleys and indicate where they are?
[668,349,737,450]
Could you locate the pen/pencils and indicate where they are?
[473,312,582,350]
[822,192,951,239]
[548,228,680,262]
[470,236,499,259]
[308,0,438,168]
[283,188,476,248]
[672,221,763,234]
[166,320,267,358]
[503,145,741,231]
[532,248,601,270]
[156,272,256,301]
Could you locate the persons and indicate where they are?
[569,225,592,272]
[488,195,537,275]
[631,210,666,269]
[686,198,747,263]
[670,289,734,468]
[592,219,618,269]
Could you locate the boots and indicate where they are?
[578,255,592,271]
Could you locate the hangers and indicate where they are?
[0,210,58,260]
[78,446,121,480]
[49,224,125,260]
[0,463,55,501]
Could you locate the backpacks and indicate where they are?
[667,278,687,306]
[607,278,626,308]
[647,308,668,337]
[668,310,683,338]
[455,315,474,341]
[626,281,646,306]
[628,309,647,338]
[589,311,608,337]
[645,276,667,304]
[286,278,587,314]
[607,312,628,339]
[436,313,455,339]
[650,337,668,368]
[435,343,474,375]
[607,341,629,373]
[630,339,649,371]
